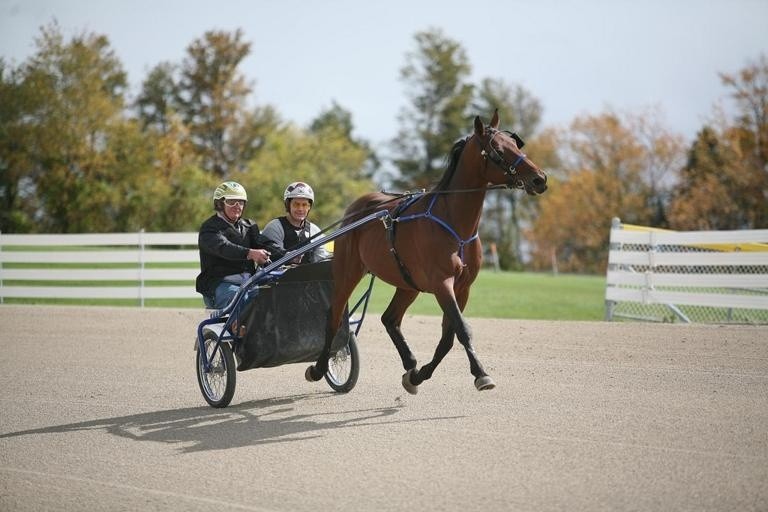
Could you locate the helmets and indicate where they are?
[283,182,314,208]
[214,182,247,206]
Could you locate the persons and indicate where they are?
[262,182,329,267]
[195,182,304,337]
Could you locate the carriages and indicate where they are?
[194,105,549,409]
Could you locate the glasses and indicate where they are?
[222,199,244,207]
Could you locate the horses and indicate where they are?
[305,108,548,394]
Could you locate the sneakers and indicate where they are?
[232,324,245,335]
